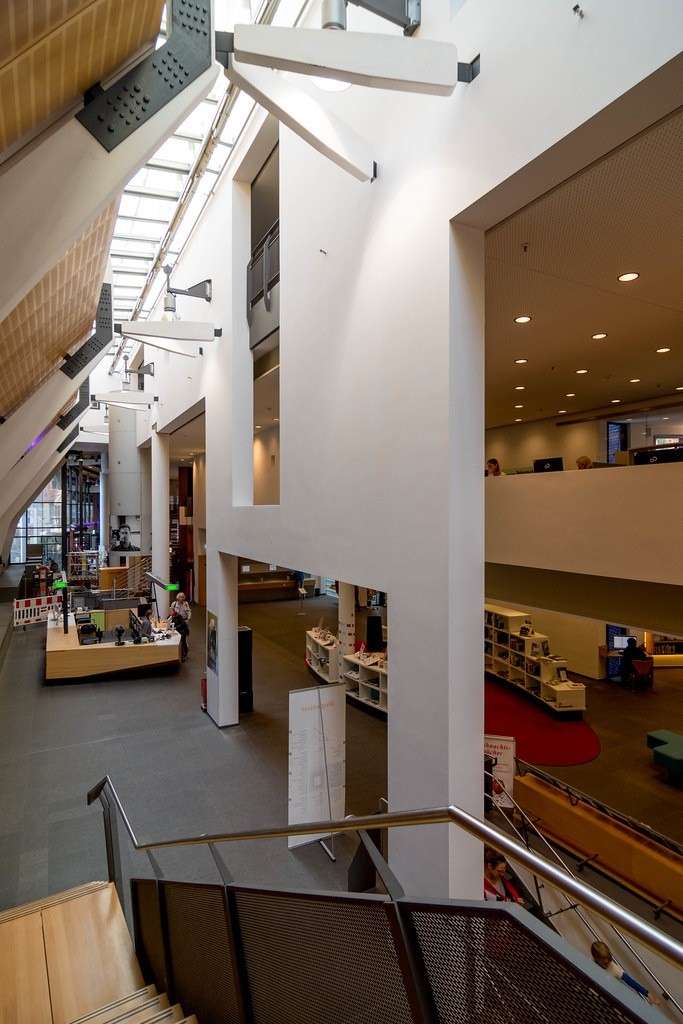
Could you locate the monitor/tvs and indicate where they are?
[613,636,636,650]
[533,457,563,473]
[164,616,175,637]
[634,448,683,465]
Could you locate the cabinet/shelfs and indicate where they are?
[483,605,586,714]
[128,608,143,635]
[303,630,340,684]
[646,632,683,667]
[344,652,389,714]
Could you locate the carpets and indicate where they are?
[484,675,601,767]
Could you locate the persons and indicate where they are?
[575,941,660,1006]
[139,610,163,639]
[294,570,305,599]
[619,638,646,690]
[487,458,506,476]
[50,560,60,574]
[167,592,191,663]
[113,524,140,551]
[576,457,592,469]
[484,845,524,1003]
[354,585,361,612]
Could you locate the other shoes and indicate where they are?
[182,655,186,660]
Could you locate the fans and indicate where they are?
[111,624,126,646]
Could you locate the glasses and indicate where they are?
[492,867,506,874]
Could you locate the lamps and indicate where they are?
[103,404,109,424]
[160,269,212,322]
[121,354,155,393]
[312,0,422,92]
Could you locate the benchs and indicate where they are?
[646,728,683,787]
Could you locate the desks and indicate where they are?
[599,644,650,683]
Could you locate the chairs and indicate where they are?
[77,618,101,645]
[628,657,655,693]
